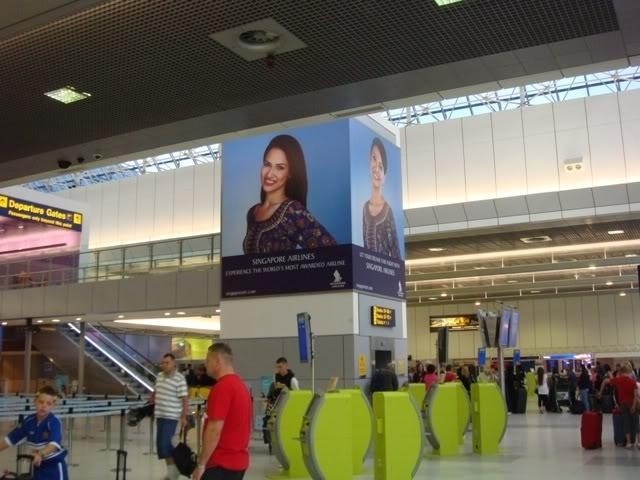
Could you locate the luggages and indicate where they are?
[612,409,636,446]
[581,412,602,449]
[1,452,35,480]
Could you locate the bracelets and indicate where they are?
[197,464,203,469]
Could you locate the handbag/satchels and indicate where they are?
[173,441,197,478]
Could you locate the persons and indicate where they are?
[408,359,640,449]
[274,358,299,390]
[150,353,189,480]
[242,134,337,254]
[363,138,401,259]
[180,364,217,386]
[0,385,68,480]
[370,361,398,393]
[190,343,251,480]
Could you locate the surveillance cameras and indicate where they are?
[58,161,71,169]
[78,157,83,163]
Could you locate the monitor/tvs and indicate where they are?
[296,312,313,363]
[477,308,521,348]
